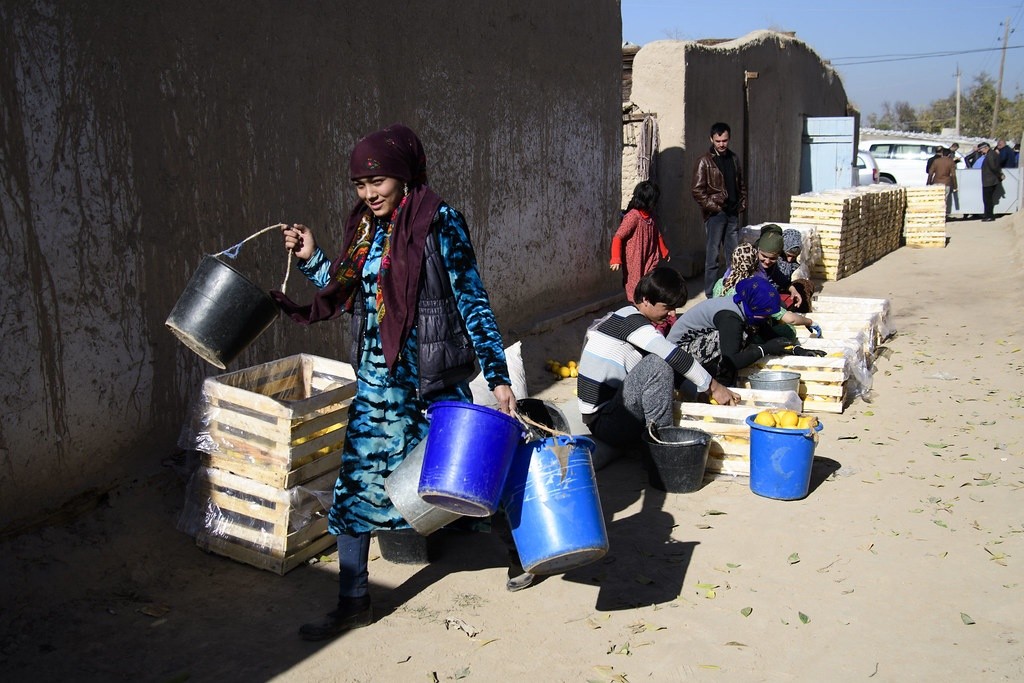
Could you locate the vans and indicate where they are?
[858,140,968,186]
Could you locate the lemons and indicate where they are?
[544,359,579,377]
[752,346,844,403]
[221,421,347,470]
[753,410,819,429]
[699,394,752,463]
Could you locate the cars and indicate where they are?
[857,151,880,187]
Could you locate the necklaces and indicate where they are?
[641,218,652,224]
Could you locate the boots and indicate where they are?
[298,530,375,642]
[491,513,536,592]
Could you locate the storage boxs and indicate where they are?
[192,353,357,576]
[742,183,947,281]
[671,296,892,476]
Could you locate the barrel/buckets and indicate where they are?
[516,398,570,433]
[418,401,525,517]
[166,224,292,370]
[747,370,801,408]
[502,434,610,574]
[643,419,713,493]
[746,413,824,501]
[383,434,461,536]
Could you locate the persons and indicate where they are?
[776,229,815,312]
[979,143,1005,222]
[577,268,741,450]
[281,124,537,640]
[666,277,827,402]
[926,146,944,173]
[964,140,1020,169]
[609,181,677,336]
[691,122,747,299]
[724,224,802,307]
[949,143,959,162]
[712,243,823,338]
[927,149,957,192]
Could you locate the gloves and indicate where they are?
[758,337,792,358]
[793,345,827,357]
[806,321,822,338]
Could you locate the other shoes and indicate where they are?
[982,217,995,221]
